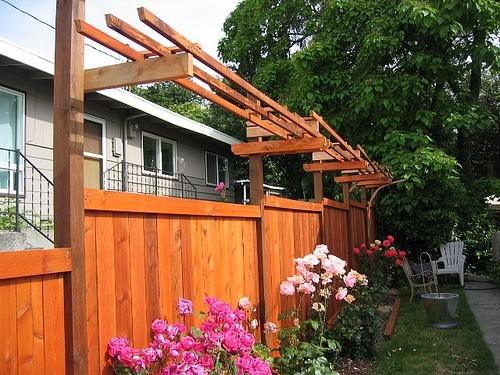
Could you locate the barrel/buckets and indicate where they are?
[421,293,459,329]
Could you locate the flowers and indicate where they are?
[277,240,369,345]
[216,181,230,202]
[353,233,407,291]
[107,290,278,374]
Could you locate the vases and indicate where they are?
[308,336,340,368]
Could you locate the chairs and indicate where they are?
[401,255,440,304]
[434,240,466,288]
